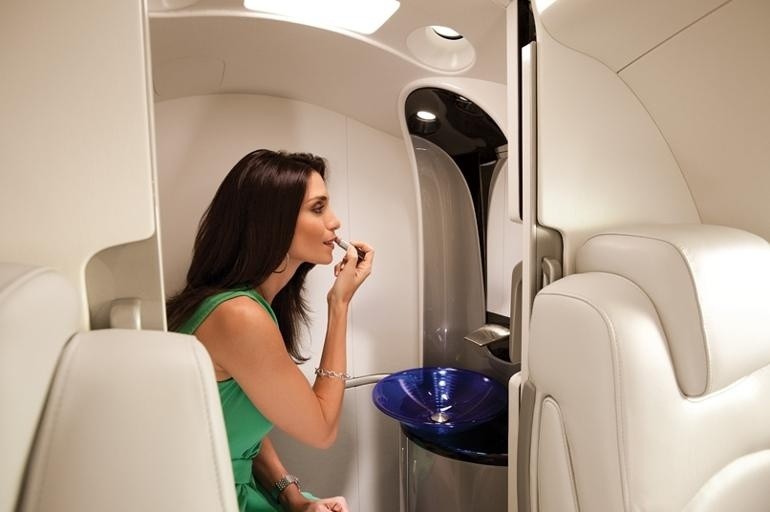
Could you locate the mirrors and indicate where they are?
[478,155,522,321]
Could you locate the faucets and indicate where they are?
[465,323,510,347]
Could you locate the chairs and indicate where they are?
[508,224,769,510]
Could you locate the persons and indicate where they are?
[166,148,375,512]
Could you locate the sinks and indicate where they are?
[372,367,507,435]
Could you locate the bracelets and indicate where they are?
[315,367,351,382]
[270,475,302,503]
